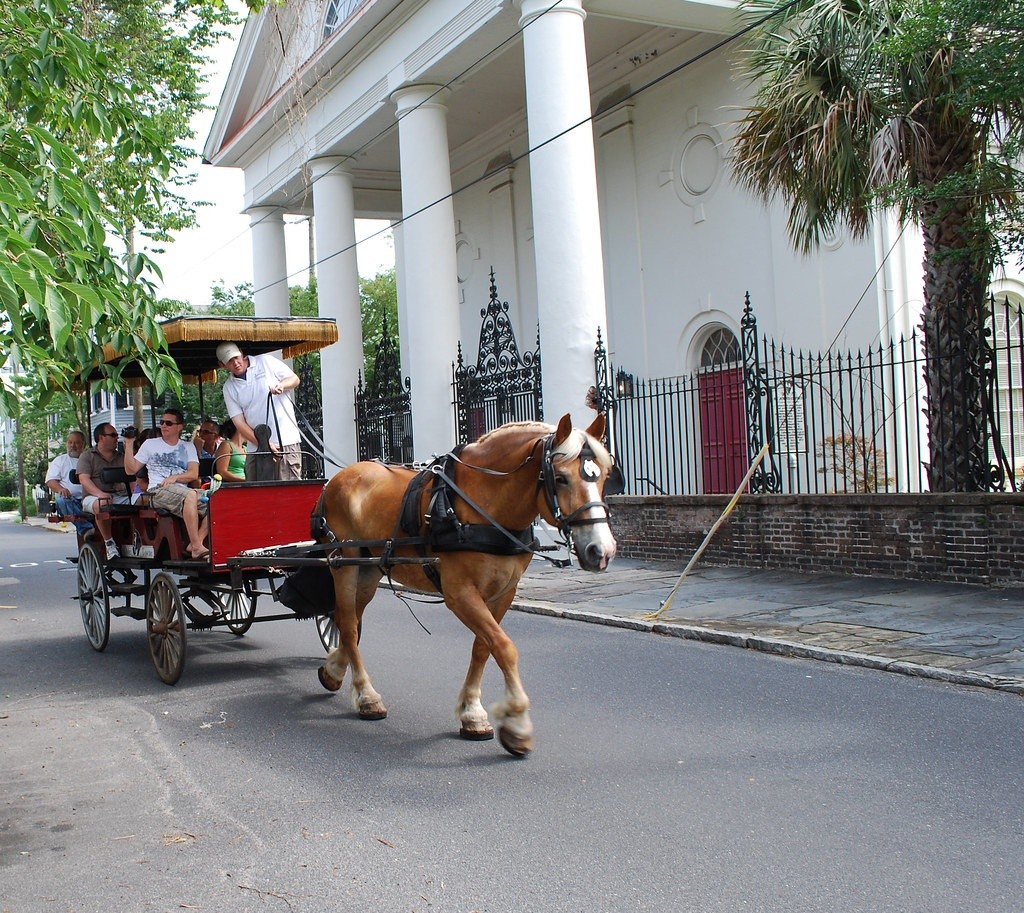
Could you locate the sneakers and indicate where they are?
[105,540,121,561]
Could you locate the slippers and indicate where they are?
[188,551,210,561]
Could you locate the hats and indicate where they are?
[216,342,242,365]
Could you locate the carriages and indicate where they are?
[47,315,618,758]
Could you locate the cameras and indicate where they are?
[121,428,138,438]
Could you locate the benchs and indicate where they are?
[48,458,216,561]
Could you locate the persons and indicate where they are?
[216,341,302,481]
[44,408,250,562]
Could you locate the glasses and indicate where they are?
[102,432,119,438]
[197,429,216,434]
[160,420,179,426]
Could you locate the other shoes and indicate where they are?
[81,527,95,541]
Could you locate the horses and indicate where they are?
[309,413,616,759]
